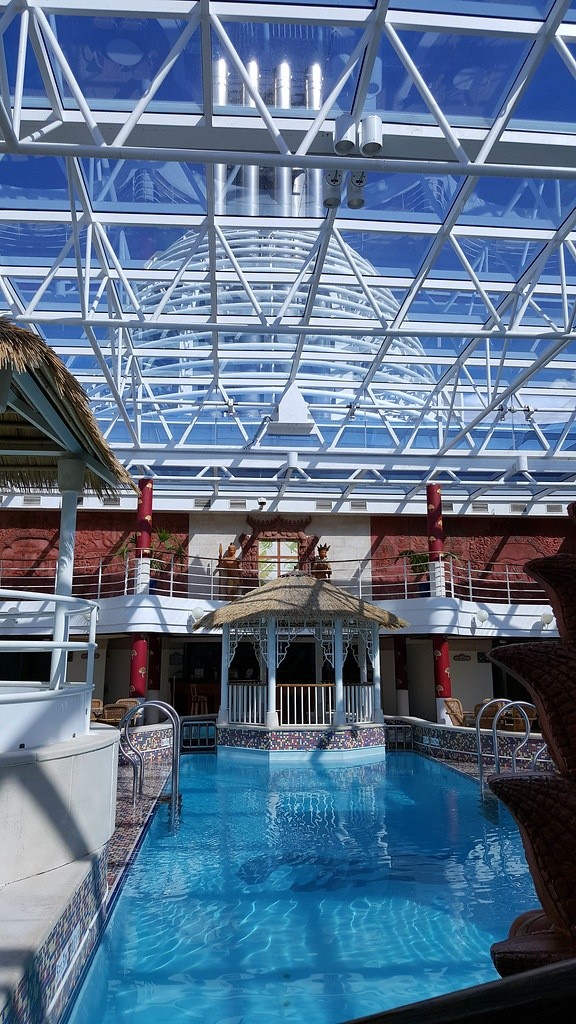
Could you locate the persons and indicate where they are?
[311,544,332,583]
[219,543,243,601]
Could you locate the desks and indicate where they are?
[94,713,143,721]
[462,709,513,724]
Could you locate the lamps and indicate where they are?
[358,115,383,157]
[346,170,367,210]
[332,111,354,156]
[320,171,343,208]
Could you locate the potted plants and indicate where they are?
[395,550,462,597]
[115,523,188,595]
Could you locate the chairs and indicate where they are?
[190,683,208,716]
[90,698,140,728]
[443,696,536,733]
